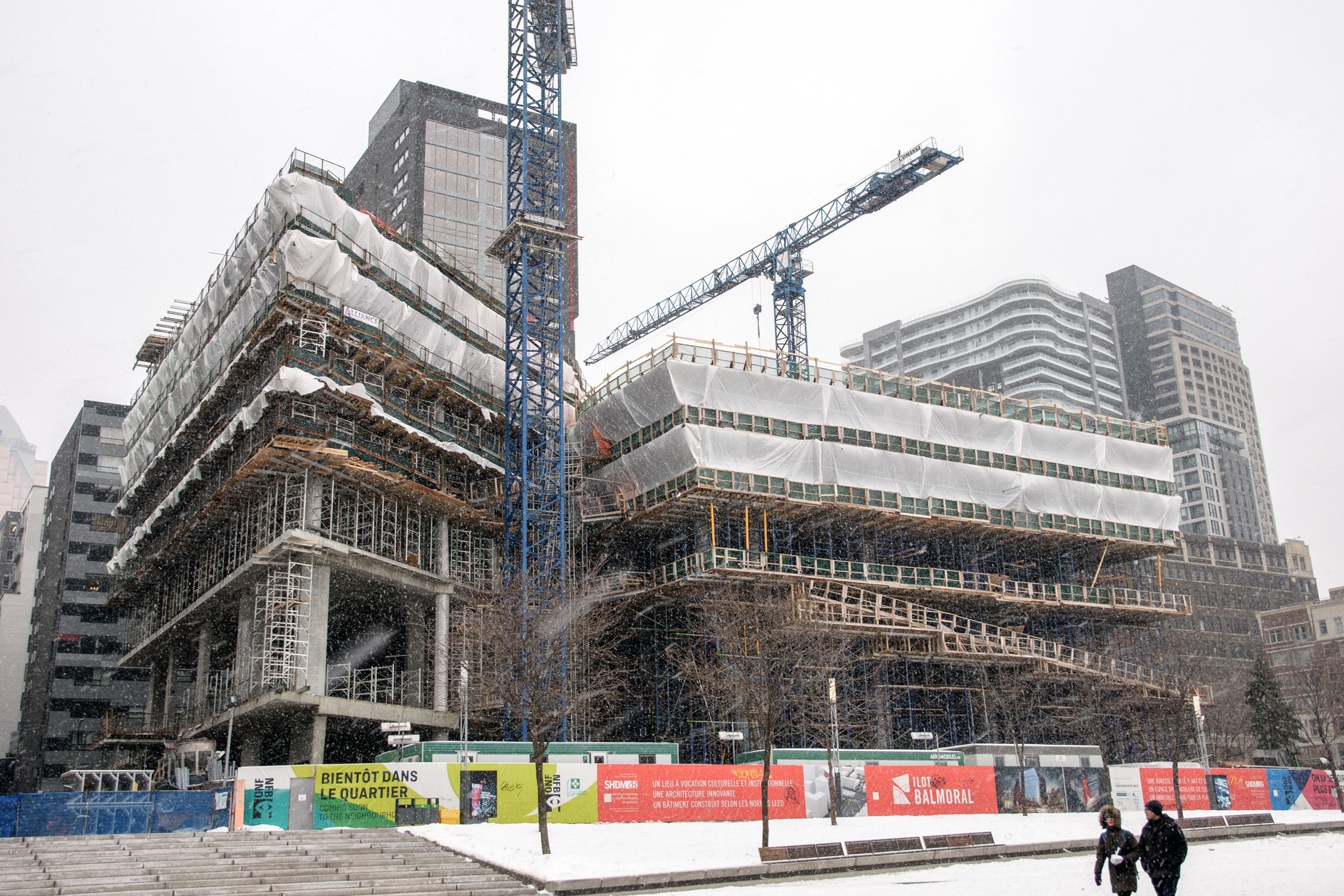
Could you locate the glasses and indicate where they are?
[1144,808,1150,811]
[1106,816,1114,819]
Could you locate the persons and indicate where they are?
[1094,805,1140,896]
[1138,800,1188,896]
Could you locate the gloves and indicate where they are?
[1095,873,1102,886]
[1116,856,1128,867]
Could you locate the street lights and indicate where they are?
[828,677,843,817]
[222,690,237,790]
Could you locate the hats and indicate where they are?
[1145,800,1163,817]
